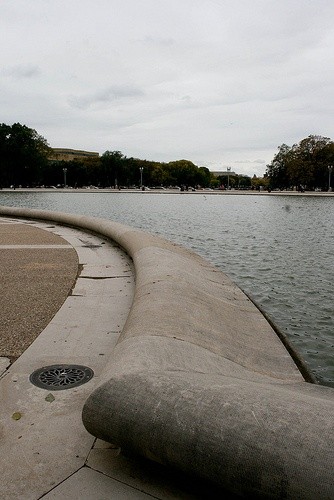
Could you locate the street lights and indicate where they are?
[328,164,333,189]
[140,166,144,186]
[63,167,67,186]
